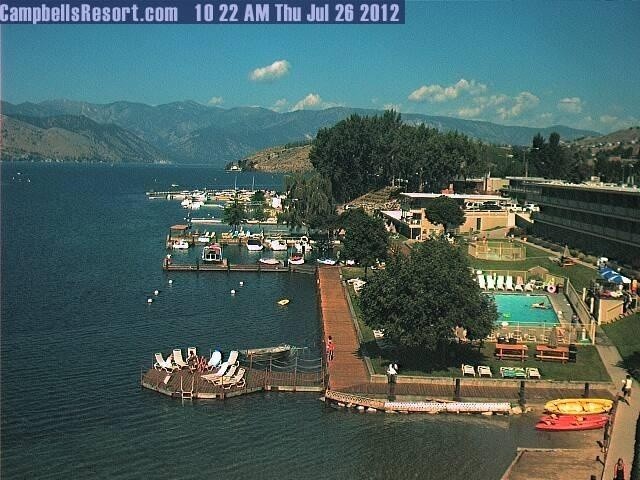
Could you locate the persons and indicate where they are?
[164,350,221,375]
[548,324,578,346]
[528,302,546,308]
[622,375,633,406]
[387,359,399,384]
[166,252,172,265]
[326,335,335,362]
[614,458,627,480]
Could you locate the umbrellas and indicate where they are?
[596,266,632,286]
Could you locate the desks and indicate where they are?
[496,343,569,364]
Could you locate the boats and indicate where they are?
[238,345,292,362]
[535,414,610,430]
[544,398,614,414]
[145,188,337,265]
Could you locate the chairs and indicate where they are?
[154,346,246,390]
[476,270,533,292]
[462,363,541,379]
